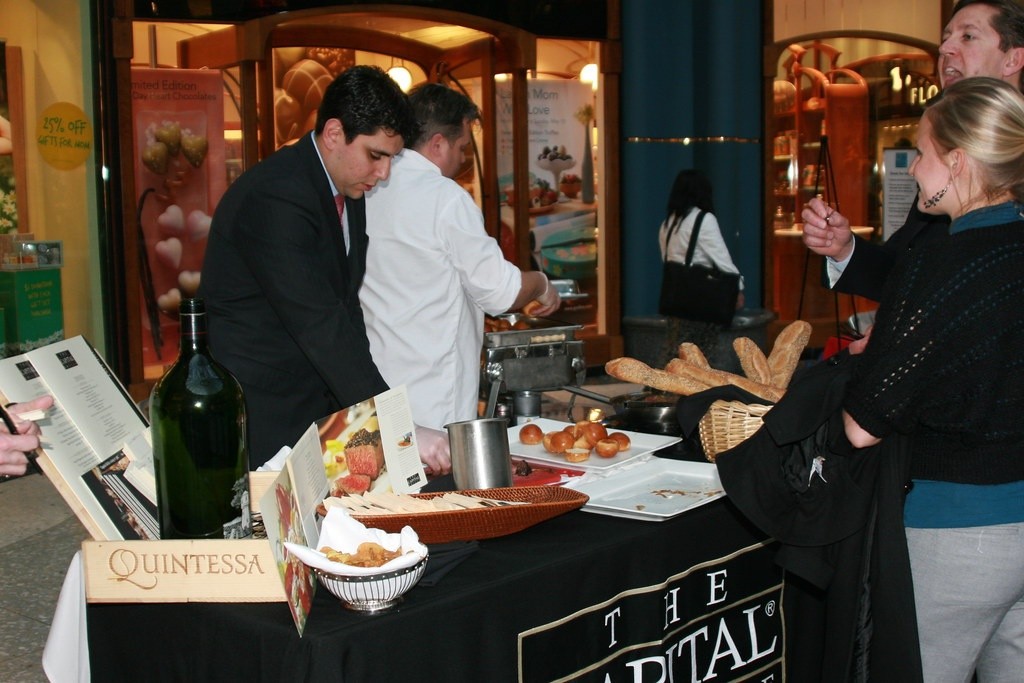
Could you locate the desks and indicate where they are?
[43,401,786,683]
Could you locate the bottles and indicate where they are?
[148,301,253,545]
[444,417,513,493]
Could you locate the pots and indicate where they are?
[561,382,691,430]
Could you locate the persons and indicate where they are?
[0,386,55,484]
[195,65,561,509]
[801,0,1024,683]
[658,168,746,375]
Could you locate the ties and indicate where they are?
[335,194,345,229]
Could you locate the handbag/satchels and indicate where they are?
[657,261,741,326]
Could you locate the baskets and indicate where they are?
[698,399,775,464]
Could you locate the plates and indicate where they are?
[556,453,733,521]
[483,418,683,475]
[509,199,557,214]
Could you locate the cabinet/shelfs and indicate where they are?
[769,42,869,338]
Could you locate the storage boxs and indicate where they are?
[80,466,287,604]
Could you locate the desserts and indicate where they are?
[270,47,354,147]
[144,122,214,317]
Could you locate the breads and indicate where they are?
[605,320,812,402]
[518,421,630,462]
